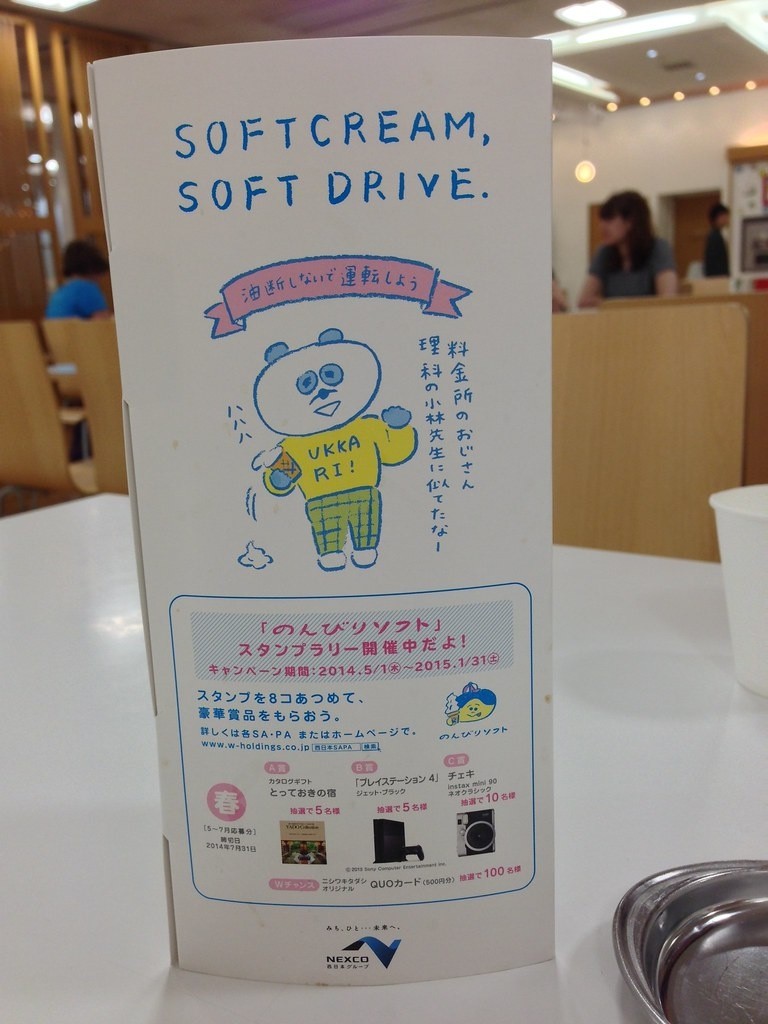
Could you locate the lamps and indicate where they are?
[552,0,627,28]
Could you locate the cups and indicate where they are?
[708,483,767,698]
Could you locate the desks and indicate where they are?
[0,491,768,1023]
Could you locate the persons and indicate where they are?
[44,239,110,321]
[702,203,730,277]
[576,191,678,309]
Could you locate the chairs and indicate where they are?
[552,299,749,564]
[0,311,130,517]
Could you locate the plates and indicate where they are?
[612,857,768,1024]
